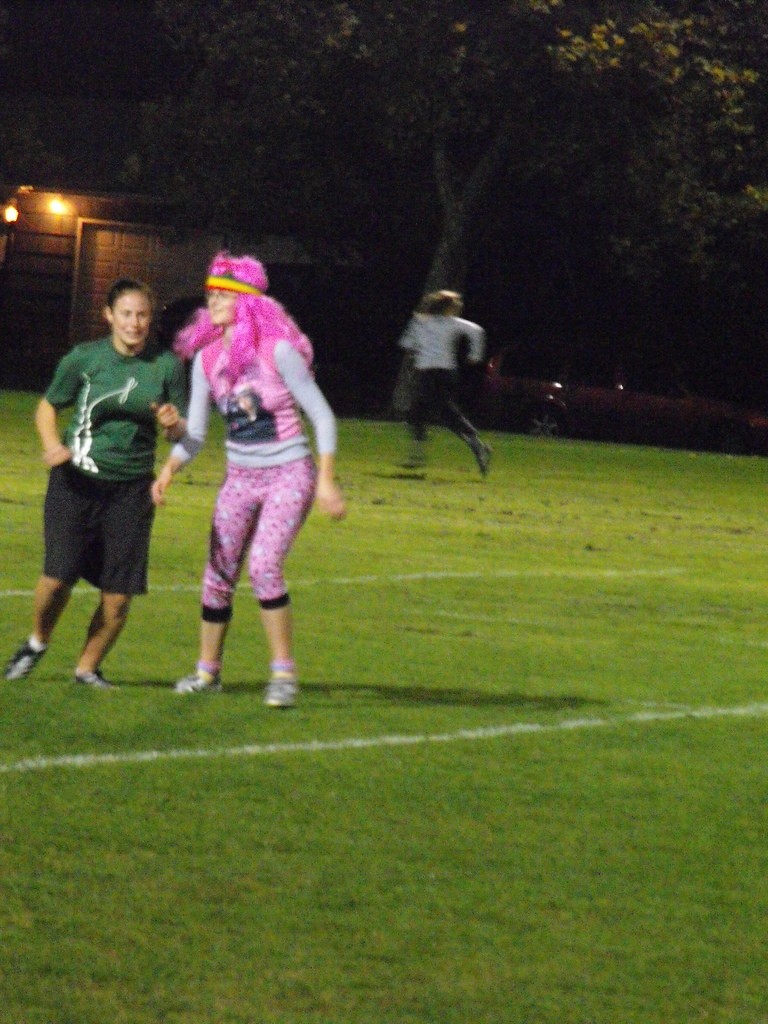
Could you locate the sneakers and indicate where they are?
[75,672,118,690]
[263,678,298,706]
[173,674,224,695]
[2,640,48,680]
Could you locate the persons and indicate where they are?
[3,281,191,688]
[399,289,495,477]
[151,252,348,708]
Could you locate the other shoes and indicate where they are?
[477,443,493,479]
